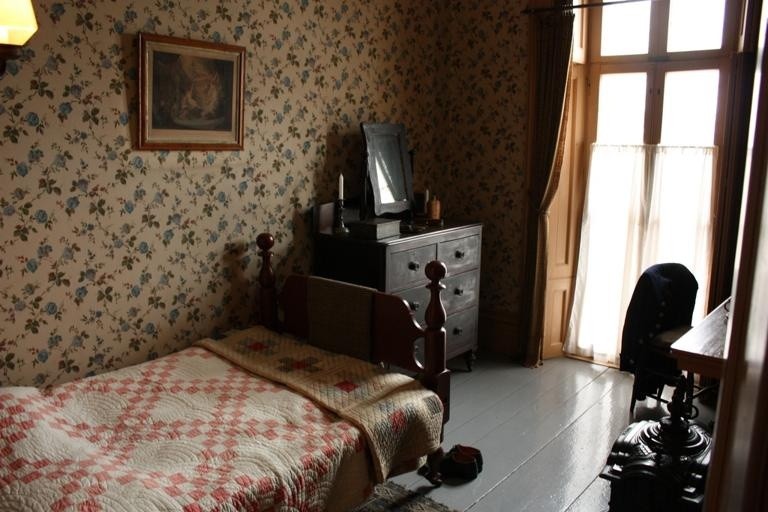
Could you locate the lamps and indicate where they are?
[0,0,38,78]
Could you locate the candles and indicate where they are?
[423,188,430,214]
[338,172,344,200]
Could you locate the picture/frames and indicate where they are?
[136,30,245,152]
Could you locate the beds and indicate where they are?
[0,232,451,512]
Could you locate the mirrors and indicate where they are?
[360,121,416,216]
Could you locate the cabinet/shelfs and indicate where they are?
[312,198,484,372]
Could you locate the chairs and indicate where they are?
[619,262,720,419]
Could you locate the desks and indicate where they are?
[669,296,732,420]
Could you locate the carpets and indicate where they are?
[357,479,457,512]
